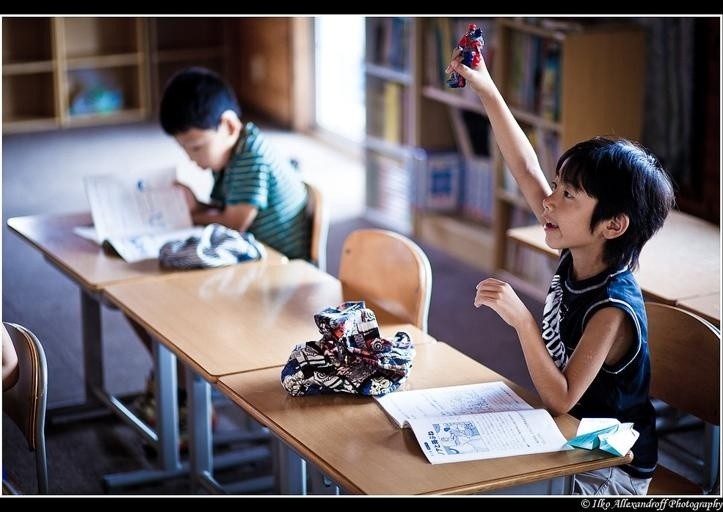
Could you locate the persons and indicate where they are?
[441,22,676,498]
[126,71,315,455]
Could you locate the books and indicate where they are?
[373,380,574,464]
[69,165,207,265]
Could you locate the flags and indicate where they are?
[446,23,484,89]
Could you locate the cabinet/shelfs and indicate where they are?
[2,14,224,137]
[359,17,643,280]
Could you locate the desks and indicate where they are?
[99,258,436,498]
[3,209,289,489]
[676,292,721,333]
[216,342,634,496]
[507,207,721,300]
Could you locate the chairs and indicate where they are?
[5,322,49,500]
[302,179,328,273]
[645,301,720,498]
[341,229,432,332]
